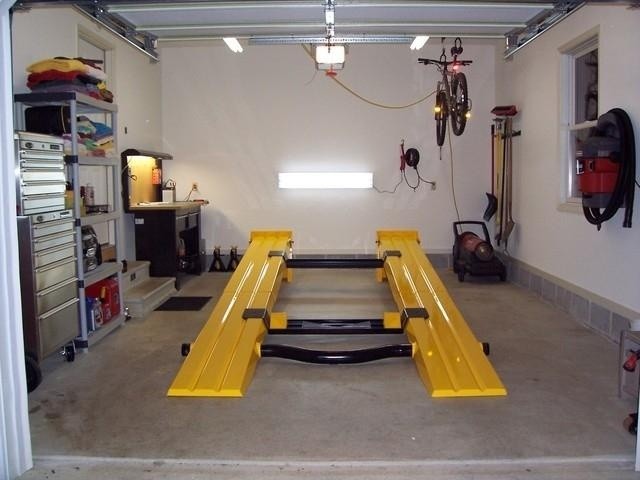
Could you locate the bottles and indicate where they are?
[80,186,87,217]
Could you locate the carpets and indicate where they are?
[154,296,212,311]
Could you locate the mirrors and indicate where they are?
[127,156,158,207]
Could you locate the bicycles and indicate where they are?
[417,57,472,146]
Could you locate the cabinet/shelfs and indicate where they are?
[16,130,82,363]
[14,90,126,355]
[135,201,209,290]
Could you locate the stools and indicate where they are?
[618,329,640,398]
